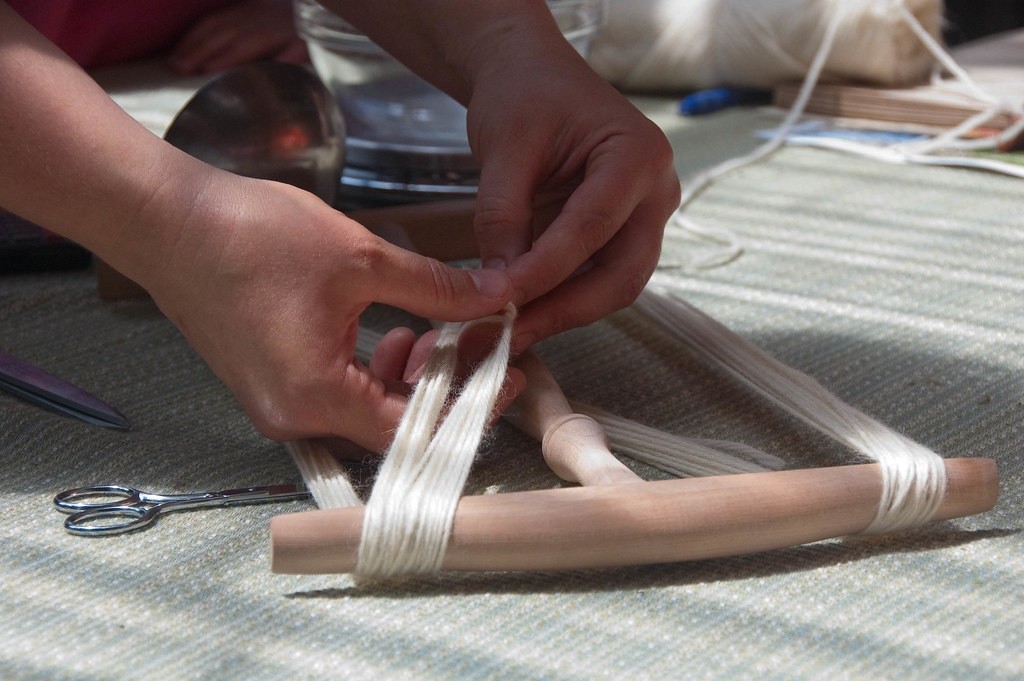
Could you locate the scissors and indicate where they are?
[0,351,130,431]
[53,484,374,536]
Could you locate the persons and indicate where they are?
[0,0,681,457]
